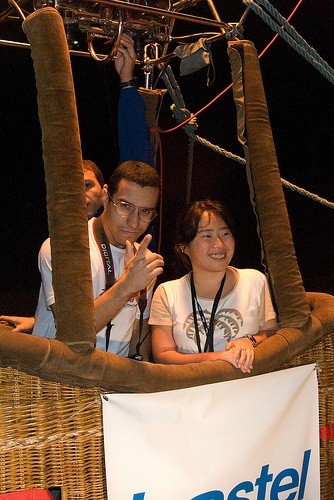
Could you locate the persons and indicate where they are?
[0,159,108,333]
[32,161,164,358]
[146,199,279,374]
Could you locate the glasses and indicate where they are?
[107,192,158,223]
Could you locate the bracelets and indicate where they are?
[247,334,258,347]
